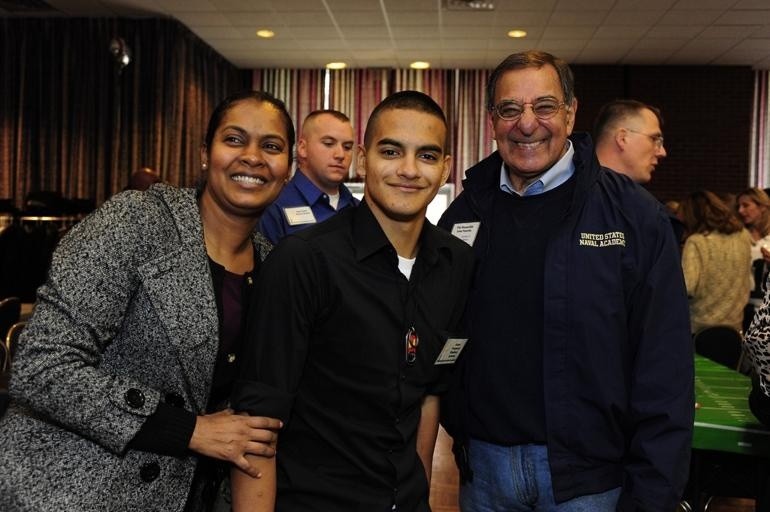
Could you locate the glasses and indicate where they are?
[626,127,663,146]
[490,99,567,122]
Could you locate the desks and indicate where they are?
[691,350,770,457]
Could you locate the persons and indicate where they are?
[227,89,474,511]
[587,98,668,183]
[434,48,696,509]
[1,89,297,511]
[257,108,363,246]
[663,182,769,423]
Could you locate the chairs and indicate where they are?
[699,491,760,512]
[6,320,30,369]
[0,297,21,343]
[693,323,746,371]
